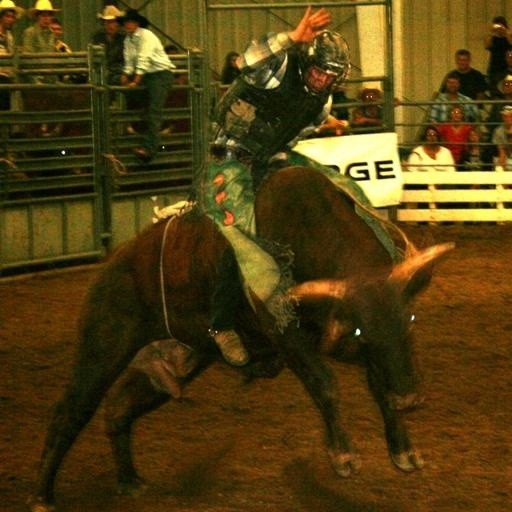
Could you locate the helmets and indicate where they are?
[296,29,350,101]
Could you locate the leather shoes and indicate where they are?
[211,327,249,366]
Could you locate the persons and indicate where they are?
[220,52,242,86]
[406,16,512,210]
[200,1,353,367]
[312,88,400,139]
[1,1,190,180]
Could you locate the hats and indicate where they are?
[0,0,26,16]
[116,8,150,28]
[96,5,126,22]
[27,0,62,17]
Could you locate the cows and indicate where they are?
[20,80,191,191]
[30,163,458,512]
